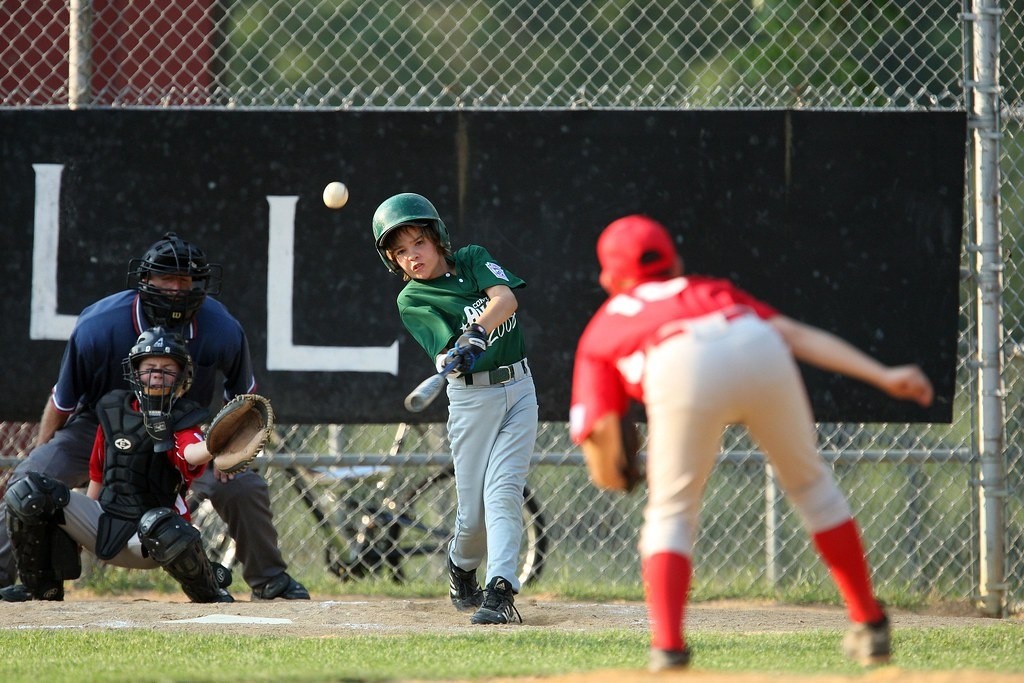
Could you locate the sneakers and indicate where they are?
[471,576,522,624]
[647,646,691,671]
[252,571,311,599]
[447,538,484,613]
[843,619,892,665]
[0,578,65,601]
[214,586,235,603]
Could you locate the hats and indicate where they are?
[595,215,675,276]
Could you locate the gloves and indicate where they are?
[442,347,475,374]
[455,323,489,358]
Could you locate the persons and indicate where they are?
[568,216,932,671]
[372,193,539,624]
[0,237,312,605]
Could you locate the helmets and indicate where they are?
[126,232,223,325]
[122,326,193,416]
[372,192,451,275]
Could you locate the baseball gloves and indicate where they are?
[203,394,270,472]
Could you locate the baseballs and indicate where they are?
[323,181,349,210]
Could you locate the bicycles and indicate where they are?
[190,424,548,587]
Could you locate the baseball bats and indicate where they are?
[404,355,462,413]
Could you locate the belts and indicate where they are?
[464,361,527,385]
